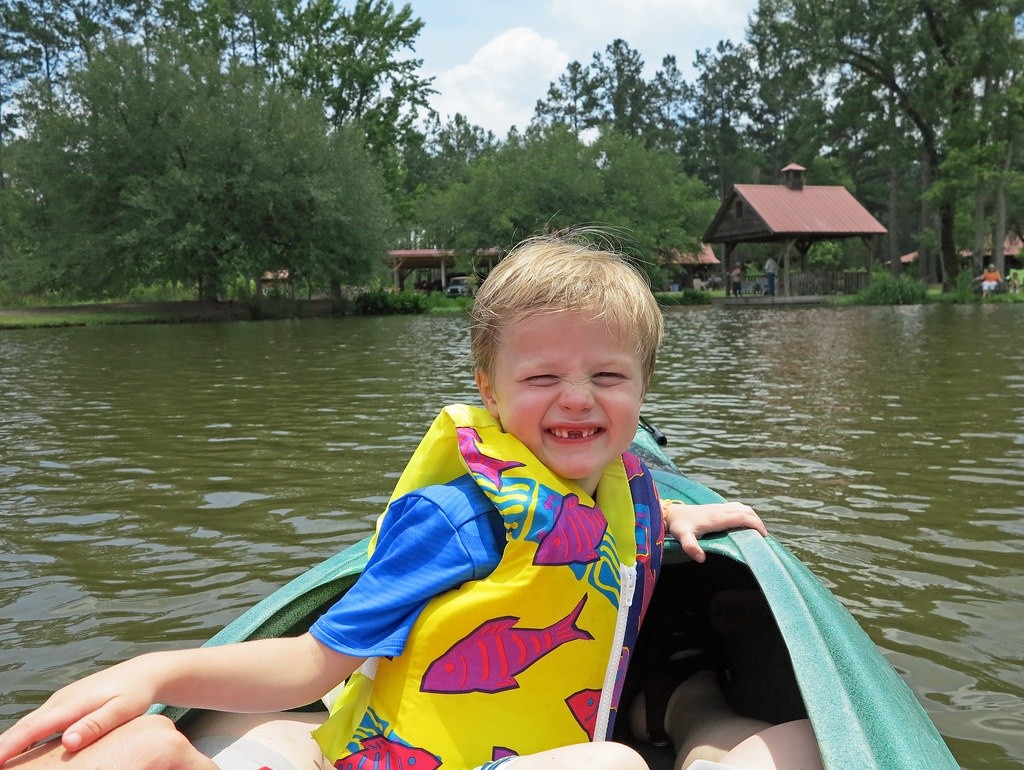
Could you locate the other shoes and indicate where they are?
[621,599,739,747]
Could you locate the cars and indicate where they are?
[447,276,478,297]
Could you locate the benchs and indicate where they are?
[973,278,1006,294]
[728,276,765,295]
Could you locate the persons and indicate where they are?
[976,264,1018,301]
[1,225,826,770]
